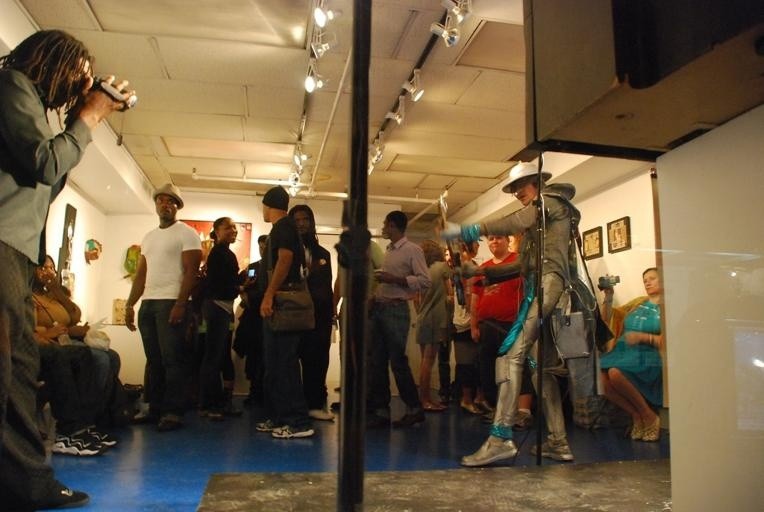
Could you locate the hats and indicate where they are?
[502,163,552,194]
[153,183,184,210]
[262,185,289,212]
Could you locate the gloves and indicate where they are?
[440,220,461,238]
[461,263,477,280]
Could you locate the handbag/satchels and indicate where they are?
[269,289,315,330]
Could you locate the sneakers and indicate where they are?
[309,408,335,421]
[461,439,518,468]
[53,429,117,456]
[272,426,314,439]
[196,407,241,420]
[630,417,660,442]
[533,440,572,461]
[132,411,183,429]
[35,483,88,508]
[256,421,275,432]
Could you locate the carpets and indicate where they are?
[197,455,669,512]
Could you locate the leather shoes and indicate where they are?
[392,411,425,427]
[460,399,492,414]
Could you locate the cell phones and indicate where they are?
[247,269,254,278]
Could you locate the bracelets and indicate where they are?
[603,298,613,304]
[649,332,654,346]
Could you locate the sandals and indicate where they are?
[424,402,446,411]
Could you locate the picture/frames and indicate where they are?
[606,216,633,254]
[581,226,603,261]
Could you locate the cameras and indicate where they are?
[90,76,136,112]
[598,275,620,288]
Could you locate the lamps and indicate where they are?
[284,0,473,196]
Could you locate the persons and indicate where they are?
[3,32,139,509]
[441,158,579,469]
[592,264,667,441]
[32,257,122,456]
[125,187,530,438]
